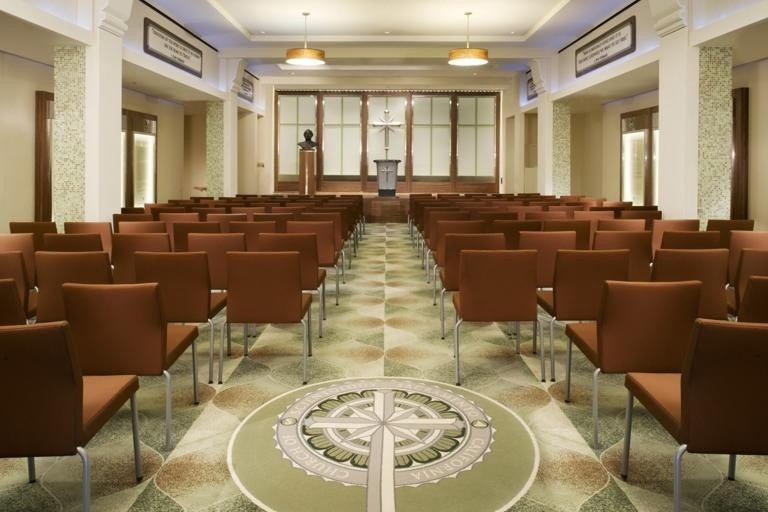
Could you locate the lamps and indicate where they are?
[447,10,489,67]
[283,10,326,68]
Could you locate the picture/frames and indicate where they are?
[572,15,637,76]
[143,17,203,77]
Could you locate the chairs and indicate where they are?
[565,280,704,449]
[452,249,547,386]
[621,318,766,509]
[63,282,200,451]
[134,251,231,383]
[533,249,631,382]
[0,191,365,326]
[218,251,314,384]
[2,321,140,512]
[405,189,767,358]
